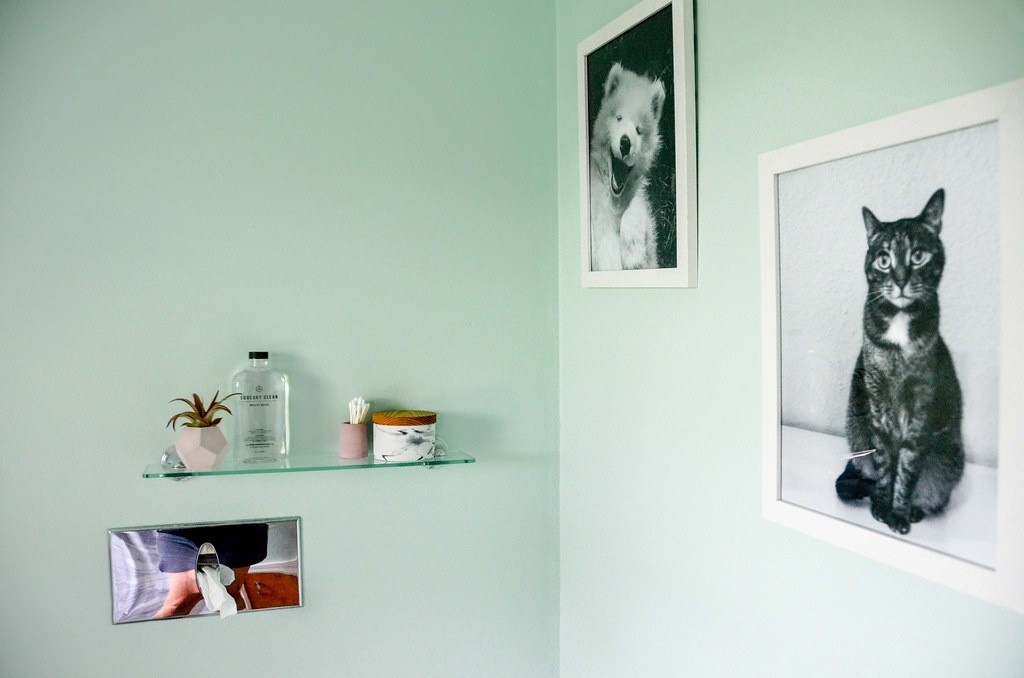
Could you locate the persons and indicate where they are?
[154,524,270,622]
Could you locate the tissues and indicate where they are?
[107,516,303,625]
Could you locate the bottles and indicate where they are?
[232,351,290,464]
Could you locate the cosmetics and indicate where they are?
[232,352,289,464]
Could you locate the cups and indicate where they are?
[338,421,369,459]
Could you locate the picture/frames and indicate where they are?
[576,0,697,289]
[757,78,1024,616]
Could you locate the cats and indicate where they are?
[836,185,966,535]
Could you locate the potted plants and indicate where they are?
[165,391,244,469]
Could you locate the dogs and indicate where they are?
[589,61,666,271]
